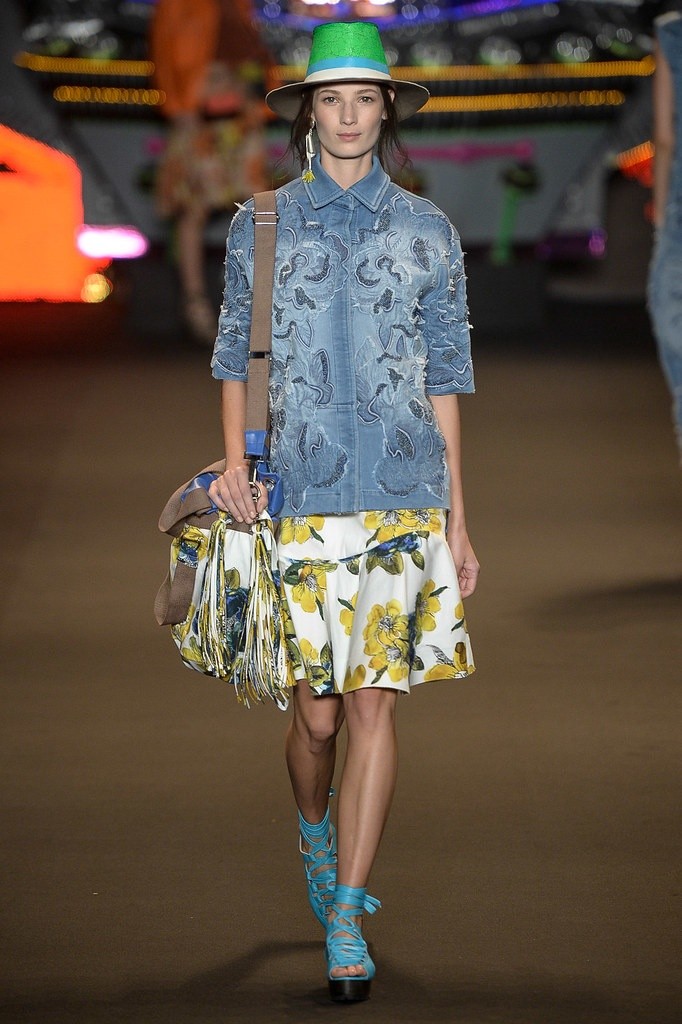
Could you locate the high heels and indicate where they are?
[298,784,383,925]
[323,883,382,1003]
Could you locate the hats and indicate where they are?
[264,20,431,125]
[200,90,245,119]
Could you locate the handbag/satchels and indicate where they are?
[136,146,162,189]
[153,455,291,707]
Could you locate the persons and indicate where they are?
[646,10,682,444]
[153,0,278,347]
[207,22,479,1005]
